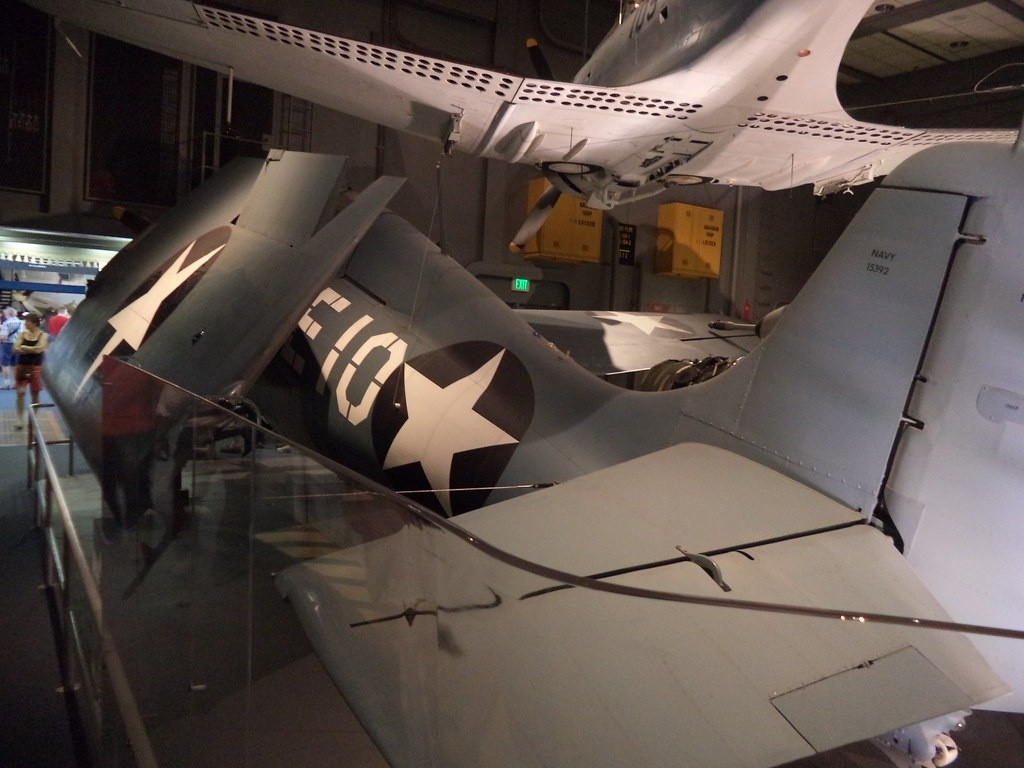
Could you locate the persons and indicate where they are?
[12,308,69,429]
[0,305,26,390]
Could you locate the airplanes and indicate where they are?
[38,141,1024,767]
[23,0,1023,258]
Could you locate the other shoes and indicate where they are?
[12,420,26,429]
[0,385,10,390]
